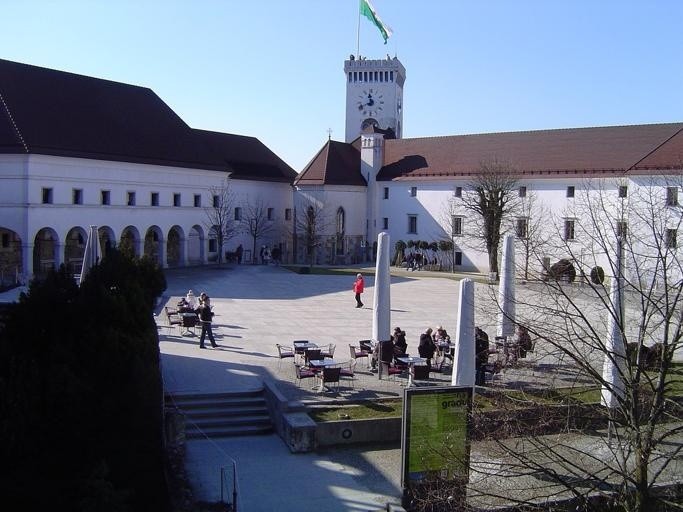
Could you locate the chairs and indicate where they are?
[276,327,541,400]
[165,303,214,337]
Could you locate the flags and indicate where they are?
[359,0,392,45]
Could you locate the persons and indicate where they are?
[199,296,218,348]
[237,244,243,264]
[271,244,280,267]
[187,290,196,310]
[197,292,210,307]
[393,327,405,339]
[352,273,363,308]
[406,249,422,272]
[368,335,393,370]
[261,245,271,264]
[418,328,436,368]
[259,245,266,264]
[392,331,405,361]
[434,326,449,357]
[509,325,531,364]
[177,297,188,306]
[474,326,488,386]
